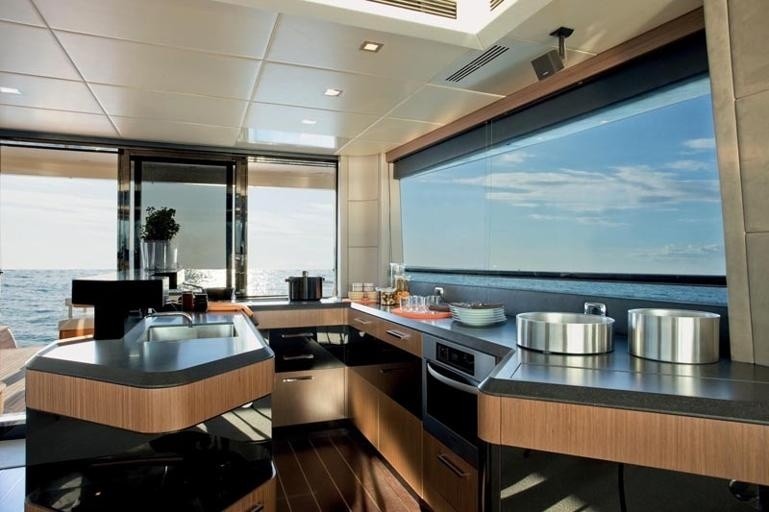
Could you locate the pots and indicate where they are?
[182,281,234,302]
[285,270,325,301]
[182,292,209,313]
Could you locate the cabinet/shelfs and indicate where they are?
[270,309,349,432]
[350,307,424,456]
[423,422,486,511]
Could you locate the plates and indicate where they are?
[448,302,508,327]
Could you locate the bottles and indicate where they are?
[351,282,374,292]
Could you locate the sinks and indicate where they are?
[628,308,720,364]
[517,312,615,356]
[137,322,238,342]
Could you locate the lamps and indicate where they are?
[531,27,579,82]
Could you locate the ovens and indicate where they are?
[421,333,495,469]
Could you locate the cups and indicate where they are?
[388,261,410,291]
[399,293,440,313]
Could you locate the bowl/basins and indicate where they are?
[348,290,378,302]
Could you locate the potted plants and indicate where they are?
[139,204,181,272]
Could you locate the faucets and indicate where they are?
[583,302,606,317]
[146,308,193,329]
